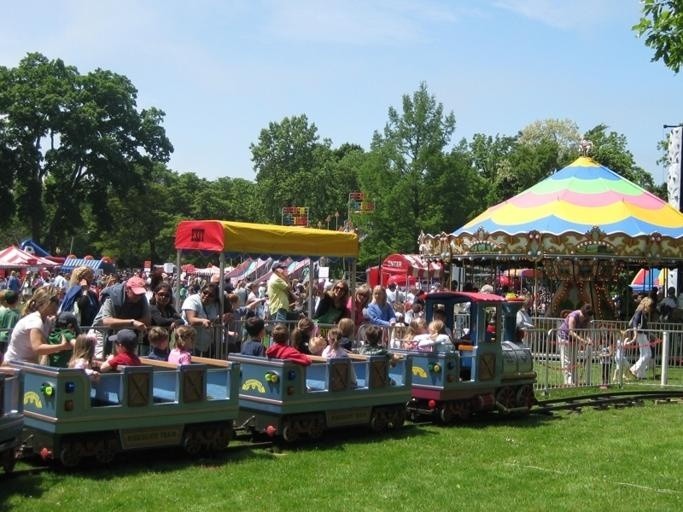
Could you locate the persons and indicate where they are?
[612,297,653,382]
[558,304,593,385]
[2,262,314,382]
[630,286,682,321]
[462,285,548,343]
[308,279,456,386]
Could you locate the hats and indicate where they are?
[272,263,286,271]
[109,329,137,348]
[126,276,146,295]
[58,311,80,333]
